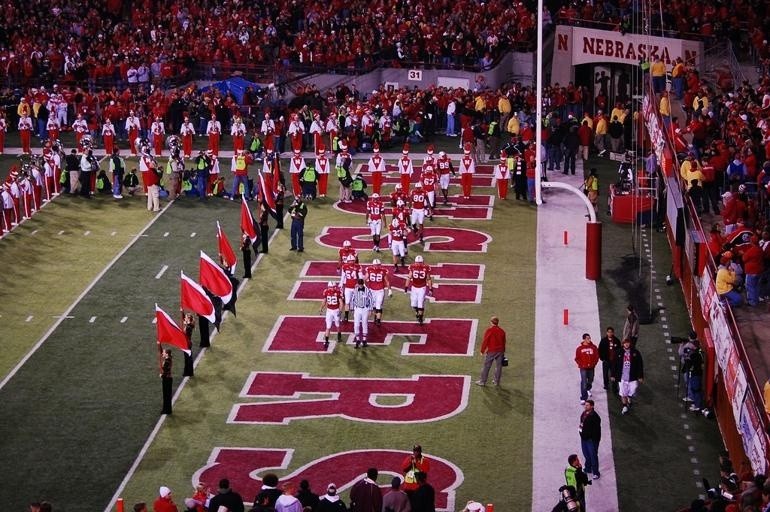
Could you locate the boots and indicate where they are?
[342,310,350,324]
[373,314,382,327]
[323,336,331,348]
[337,331,342,342]
[411,305,425,324]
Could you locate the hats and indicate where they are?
[0,145,59,205]
[412,444,421,452]
[2,1,770,140]
[718,182,760,260]
[326,482,337,491]
[159,487,172,499]
[200,143,506,166]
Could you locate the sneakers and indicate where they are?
[622,404,633,416]
[338,198,353,203]
[474,380,487,387]
[683,395,701,413]
[463,195,470,199]
[593,474,599,480]
[0,191,61,240]
[586,390,593,397]
[354,339,368,349]
[580,399,586,405]
[492,378,499,387]
[288,248,304,253]
[113,192,125,200]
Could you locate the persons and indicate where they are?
[584,168,599,216]
[0,1,769,188]
[551,305,646,512]
[29,498,52,512]
[318,219,432,349]
[678,332,706,412]
[679,138,770,309]
[681,450,769,512]
[259,193,308,253]
[0,139,550,240]
[134,442,487,512]
[476,317,506,385]
[156,227,255,415]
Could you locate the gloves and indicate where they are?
[337,310,343,317]
[387,289,393,297]
[319,307,324,315]
[428,289,433,296]
[404,287,409,294]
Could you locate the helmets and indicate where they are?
[327,281,338,287]
[371,192,380,199]
[394,182,424,207]
[426,151,447,172]
[414,255,424,264]
[342,240,352,247]
[392,218,400,228]
[372,258,381,266]
